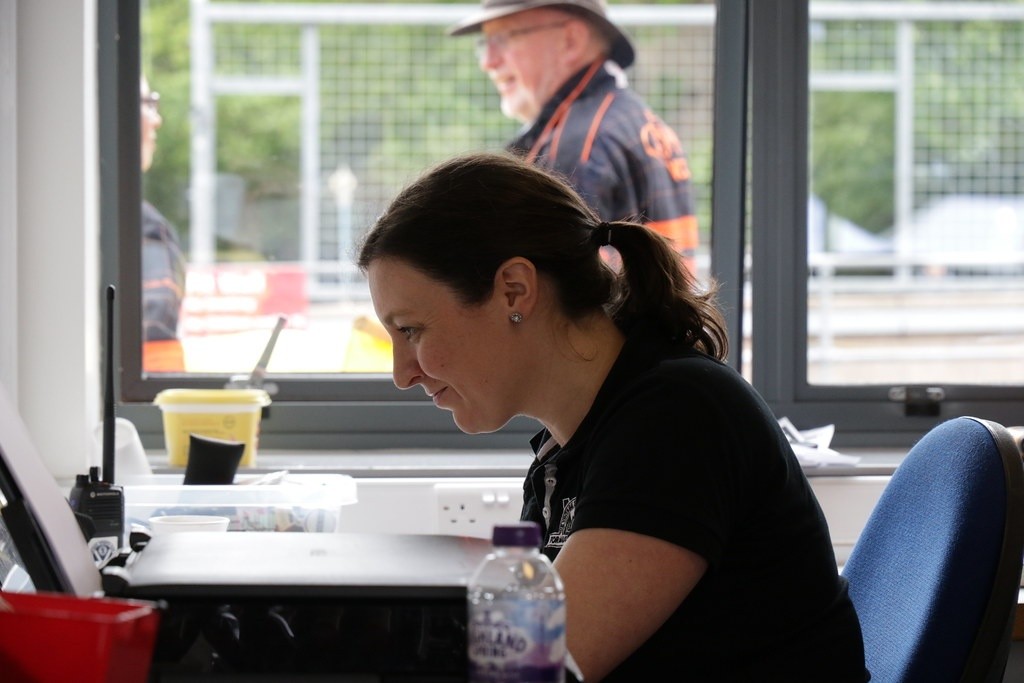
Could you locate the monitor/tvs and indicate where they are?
[0,405,103,601]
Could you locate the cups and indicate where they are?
[150,516,230,534]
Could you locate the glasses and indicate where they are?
[139,91,160,108]
[477,18,570,48]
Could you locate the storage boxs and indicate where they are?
[122,474,359,534]
[153,386,271,470]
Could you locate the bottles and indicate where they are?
[468,522,567,683]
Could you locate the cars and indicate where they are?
[806,176,1024,282]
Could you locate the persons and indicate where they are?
[140,74,186,371]
[359,154,872,683]
[448,0,697,289]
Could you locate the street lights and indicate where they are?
[330,166,357,308]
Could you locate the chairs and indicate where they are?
[845,414,1024,683]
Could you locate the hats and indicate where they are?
[450,0,635,70]
